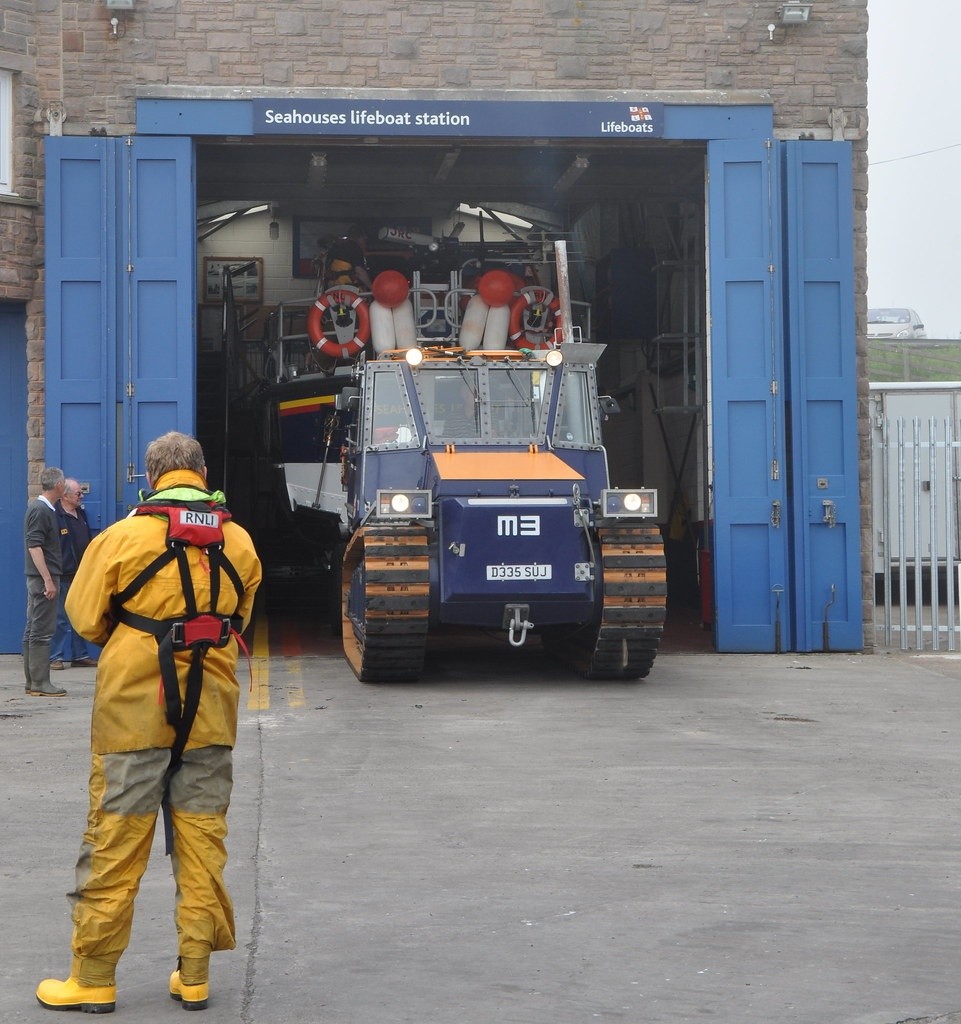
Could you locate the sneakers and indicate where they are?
[50,661,65,670]
[71,658,98,667]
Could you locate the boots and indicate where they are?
[22,643,66,696]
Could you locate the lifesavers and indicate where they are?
[510,288,565,353]
[306,286,369,359]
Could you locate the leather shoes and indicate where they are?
[169,969,209,1011]
[35,974,116,1015]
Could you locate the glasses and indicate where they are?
[67,492,82,497]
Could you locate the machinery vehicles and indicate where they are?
[275,270,667,684]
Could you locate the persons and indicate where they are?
[37,431,262,1014]
[22,466,67,697]
[324,227,373,292]
[50,478,97,670]
